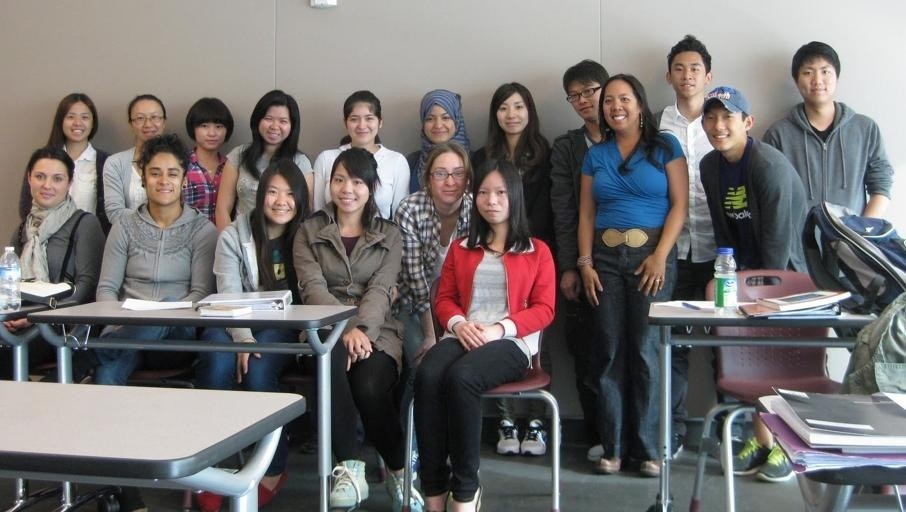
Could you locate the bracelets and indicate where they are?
[578,256,594,270]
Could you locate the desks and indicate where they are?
[27,297,361,512]
[645,298,880,510]
[0,294,79,511]
[0,368,305,512]
[760,382,905,510]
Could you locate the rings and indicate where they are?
[655,278,661,284]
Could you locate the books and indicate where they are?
[757,288,851,310]
[842,446,906,456]
[771,386,906,449]
[740,303,842,321]
[197,303,253,318]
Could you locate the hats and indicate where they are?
[703,86,751,116]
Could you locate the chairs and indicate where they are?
[398,321,565,511]
[689,270,848,511]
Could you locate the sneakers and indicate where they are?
[520,418,549,458]
[711,432,798,484]
[496,419,520,456]
[670,436,685,460]
[383,465,424,512]
[328,459,371,508]
[116,486,148,512]
[592,455,631,477]
[639,457,666,477]
[72,350,92,384]
[587,444,605,462]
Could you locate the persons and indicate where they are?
[94,133,216,512]
[195,160,310,511]
[701,85,812,482]
[1,145,105,382]
[650,33,736,459]
[103,94,170,226]
[19,94,112,240]
[413,158,558,511]
[548,60,612,462]
[179,96,238,227]
[577,71,689,478]
[405,88,477,193]
[215,89,314,235]
[763,40,892,250]
[312,91,411,223]
[474,82,559,456]
[392,143,475,480]
[291,147,424,511]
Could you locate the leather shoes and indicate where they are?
[258,472,288,509]
[195,491,224,512]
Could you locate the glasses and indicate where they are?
[566,86,602,103]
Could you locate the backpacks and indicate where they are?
[801,200,906,316]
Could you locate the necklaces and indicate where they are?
[202,164,217,174]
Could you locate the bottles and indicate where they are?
[0,244,22,314]
[713,248,739,314]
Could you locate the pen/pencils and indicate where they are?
[682,303,700,311]
